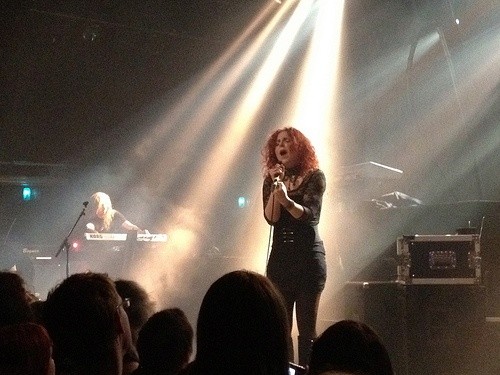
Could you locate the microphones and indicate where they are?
[273,173,281,187]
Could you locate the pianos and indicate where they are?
[71,231,168,255]
[327,160,404,181]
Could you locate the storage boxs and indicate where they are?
[397,233,482,286]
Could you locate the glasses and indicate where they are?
[116,298,131,311]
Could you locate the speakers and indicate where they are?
[363,281,486,375]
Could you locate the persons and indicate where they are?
[263,128,327,375]
[1,269,195,375]
[185,270,292,375]
[86,191,151,235]
[309,319,394,375]
